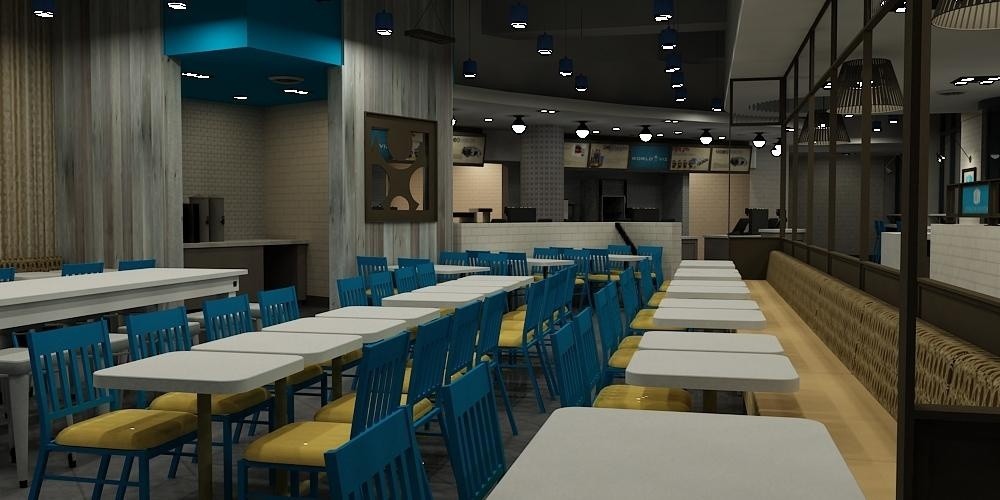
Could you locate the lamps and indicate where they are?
[887,115,899,124]
[831,57,904,115]
[770,143,781,157]
[375,0,723,112]
[885,154,900,175]
[930,0,1000,31]
[575,121,591,139]
[699,129,713,145]
[639,125,653,143]
[510,114,528,135]
[774,137,781,152]
[872,115,882,132]
[798,109,851,147]
[752,132,766,148]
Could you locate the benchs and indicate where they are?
[736,251,1000,500]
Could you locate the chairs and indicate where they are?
[0,244,667,500]
[874,220,886,264]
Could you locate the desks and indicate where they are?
[485,407,867,500]
[625,257,799,414]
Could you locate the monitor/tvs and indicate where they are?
[731,219,749,234]
